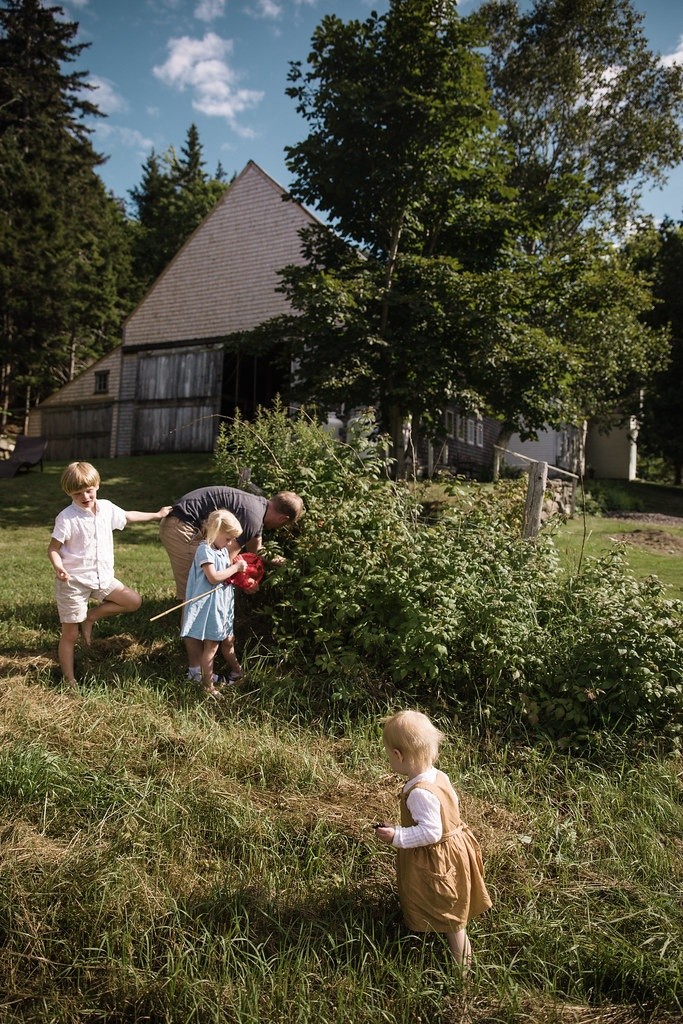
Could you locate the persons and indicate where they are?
[180,510,250,701]
[49,462,173,696]
[159,486,303,683]
[376,710,492,979]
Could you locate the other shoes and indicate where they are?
[202,688,225,702]
[228,668,246,680]
[188,667,218,685]
